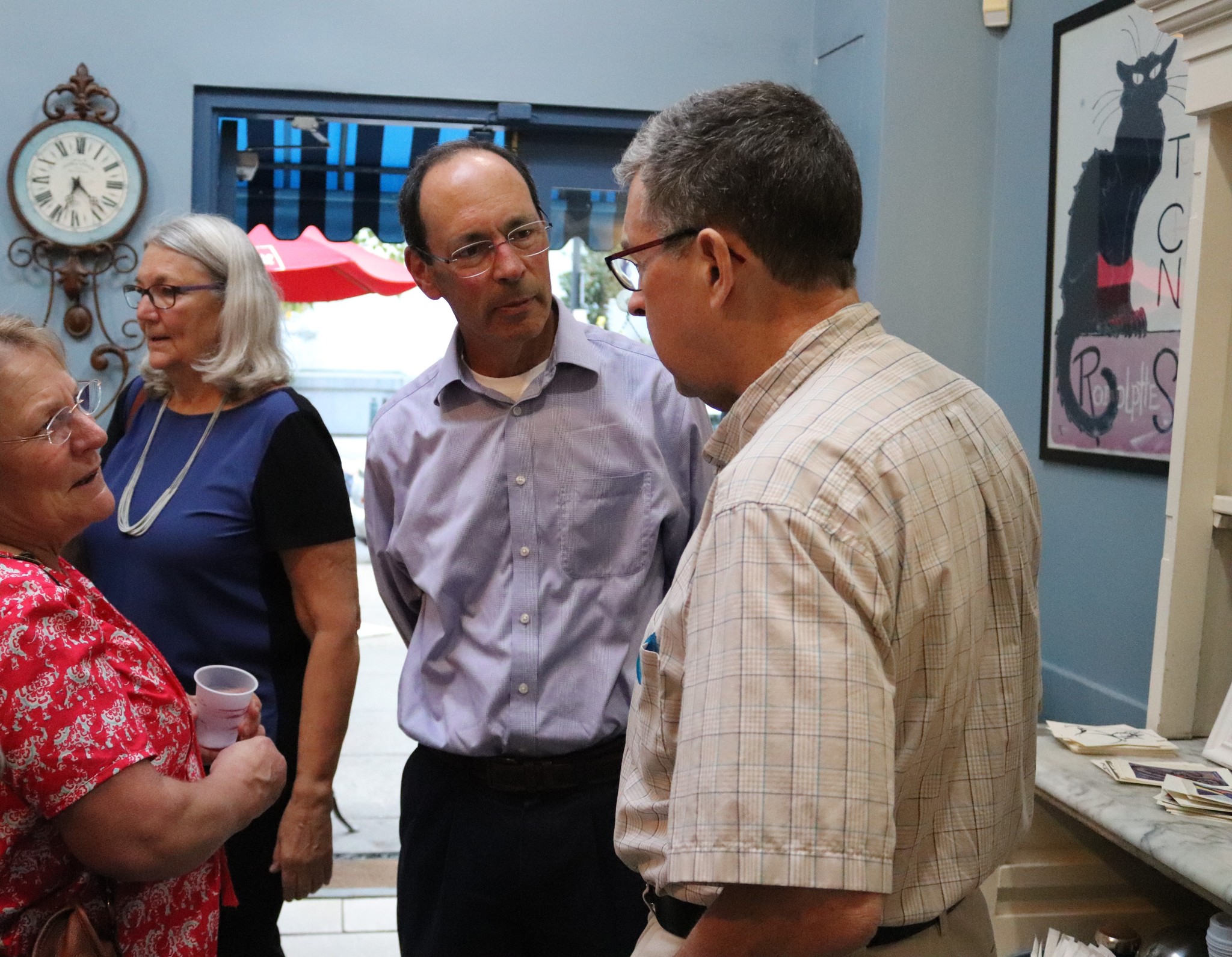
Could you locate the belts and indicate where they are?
[647,891,969,947]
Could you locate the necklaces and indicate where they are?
[0,548,62,588]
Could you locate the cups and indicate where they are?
[193,665,259,752]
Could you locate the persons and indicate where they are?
[604,79,1045,957]
[0,311,288,957]
[73,209,363,957]
[361,135,720,957]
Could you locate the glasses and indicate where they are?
[415,203,553,279]
[122,283,225,310]
[0,379,102,447]
[605,226,747,292]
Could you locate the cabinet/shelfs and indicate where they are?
[979,0,1232,957]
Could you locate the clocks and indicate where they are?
[6,62,147,420]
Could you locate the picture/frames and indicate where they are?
[1038,0,1196,476]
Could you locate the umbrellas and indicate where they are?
[248,224,417,303]
